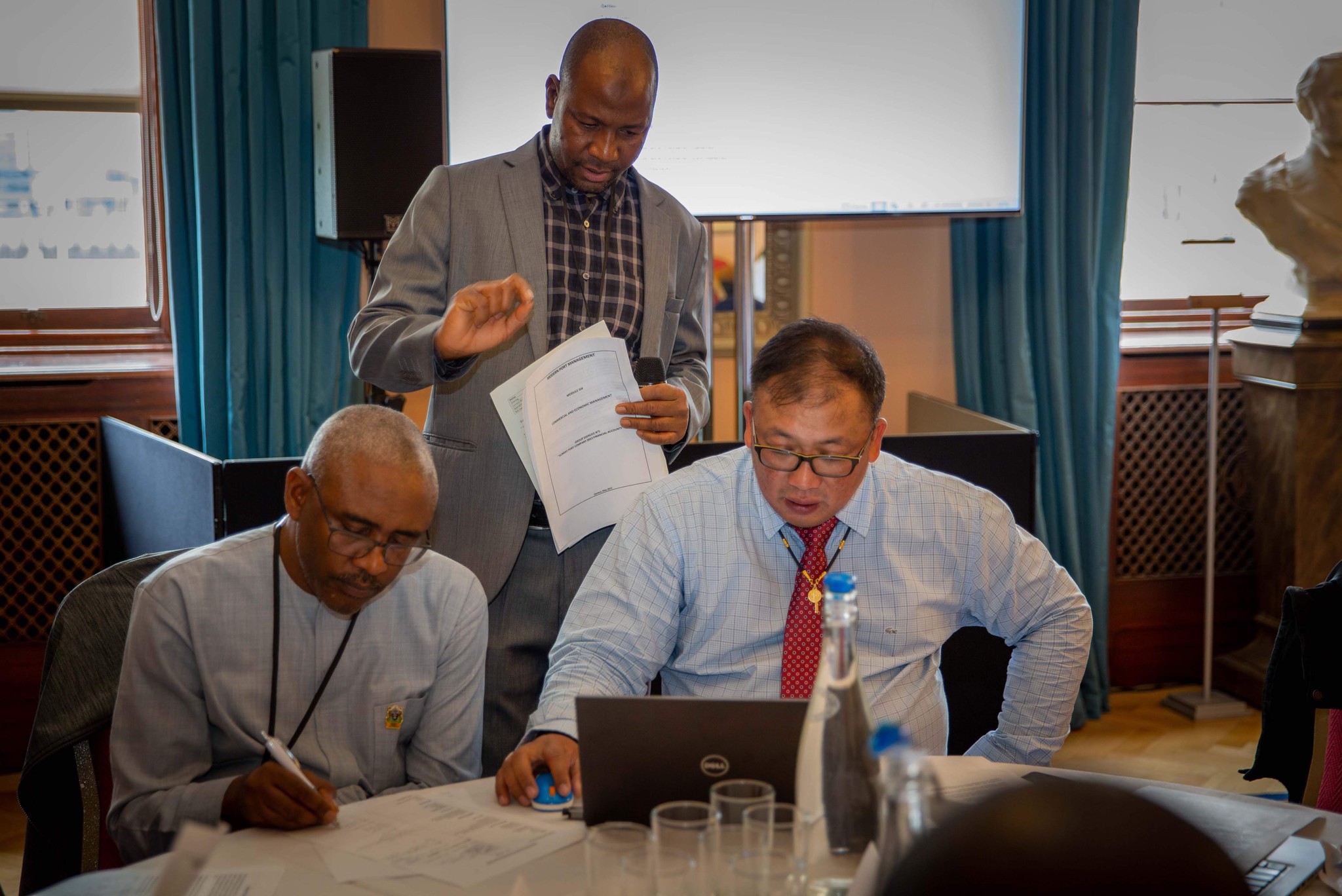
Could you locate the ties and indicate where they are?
[778,517,840,699]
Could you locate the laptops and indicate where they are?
[575,697,810,831]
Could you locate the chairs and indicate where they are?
[16,545,199,896]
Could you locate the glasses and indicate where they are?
[307,471,432,566]
[751,408,875,478]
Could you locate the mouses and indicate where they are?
[531,772,574,811]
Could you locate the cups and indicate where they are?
[622,846,690,896]
[585,822,654,896]
[735,849,790,896]
[651,801,722,896]
[699,824,769,896]
[708,780,775,896]
[742,803,794,896]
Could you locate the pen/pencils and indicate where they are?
[259,729,341,829]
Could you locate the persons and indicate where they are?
[497,318,1094,805]
[1237,52,1342,316]
[105,404,488,869]
[348,19,710,777]
[873,779,1257,896]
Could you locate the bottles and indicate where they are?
[794,572,873,896]
[847,722,914,896]
[879,751,934,896]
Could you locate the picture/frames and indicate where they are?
[703,220,805,358]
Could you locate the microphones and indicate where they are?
[632,356,668,392]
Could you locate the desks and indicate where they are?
[27,756,1342,896]
